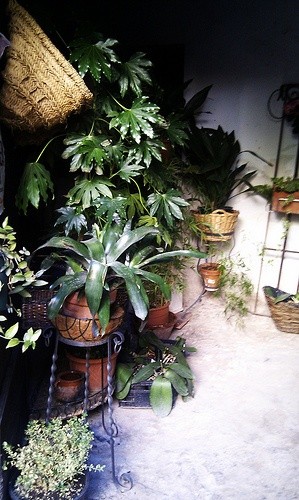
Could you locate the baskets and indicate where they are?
[20,289,59,331]
[263,278,299,334]
[0,0,94,132]
[189,210,239,241]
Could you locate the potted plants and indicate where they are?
[59,341,120,397]
[3,408,103,500]
[272,176,299,215]
[30,212,209,334]
[176,125,256,242]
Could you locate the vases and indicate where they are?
[113,331,196,417]
[55,369,84,402]
[197,263,225,292]
